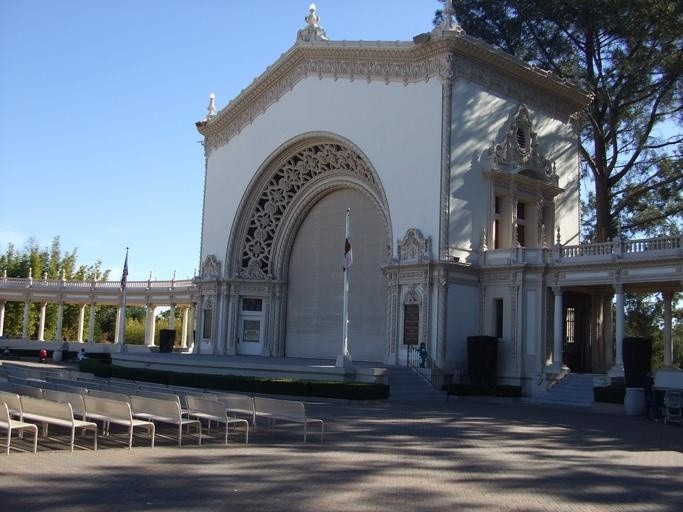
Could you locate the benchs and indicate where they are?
[0,362,325,455]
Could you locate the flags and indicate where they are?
[119,249,129,290]
[341,212,354,270]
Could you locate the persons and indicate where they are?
[0,346,10,359]
[419,342,427,368]
[643,371,659,423]
[39,348,48,364]
[76,347,84,360]
[58,338,69,362]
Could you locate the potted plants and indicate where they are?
[623,337,652,416]
